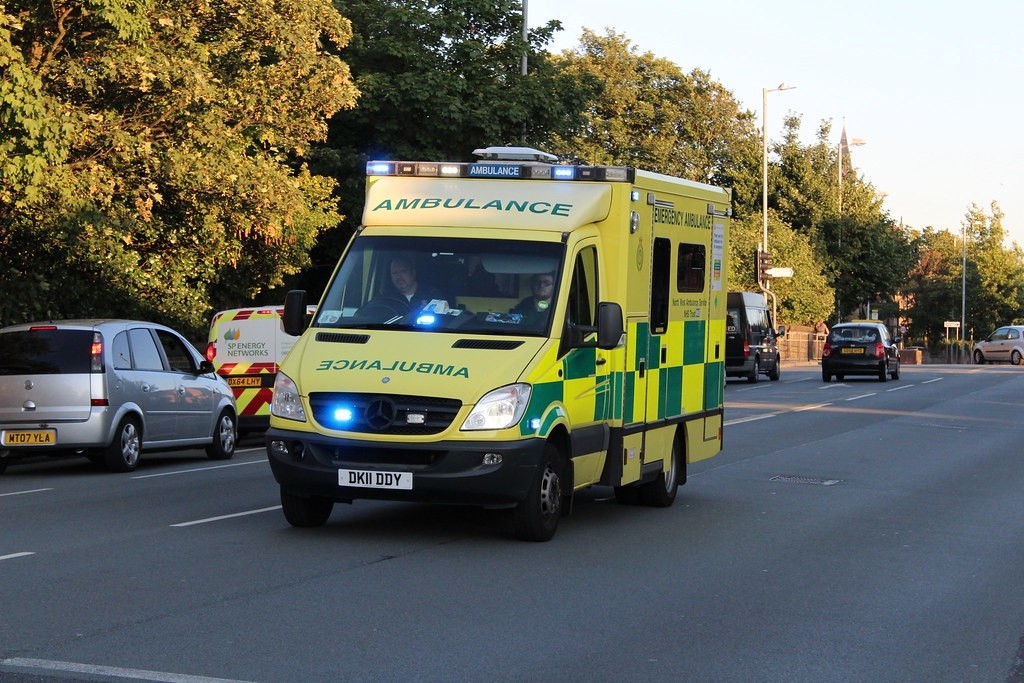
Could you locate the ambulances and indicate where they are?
[263,147,734,540]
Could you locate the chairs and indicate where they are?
[412,251,449,296]
[842,330,852,338]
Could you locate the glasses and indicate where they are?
[533,279,553,287]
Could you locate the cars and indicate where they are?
[973,325,1024,365]
[822,320,902,382]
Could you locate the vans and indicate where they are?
[725,292,786,382]
[207,304,317,434]
[0,319,239,470]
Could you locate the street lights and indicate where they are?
[837,139,866,324]
[763,83,793,304]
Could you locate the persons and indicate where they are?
[516,273,554,312]
[379,258,443,315]
